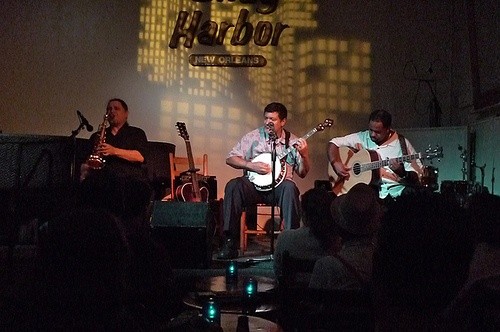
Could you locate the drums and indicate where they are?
[440,179,492,202]
[411,166,440,191]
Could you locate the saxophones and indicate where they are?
[87,111,109,172]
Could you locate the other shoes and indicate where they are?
[216,238,239,259]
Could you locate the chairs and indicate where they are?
[239,168,286,256]
[170,152,209,201]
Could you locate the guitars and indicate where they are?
[326,145,444,197]
[248,118,335,192]
[173,120,212,204]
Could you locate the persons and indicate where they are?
[0,167,223,332]
[328,109,423,199]
[80,97,152,223]
[217,102,311,259]
[274,183,500,332]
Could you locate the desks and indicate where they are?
[182,275,284,332]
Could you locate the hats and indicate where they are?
[329,183,385,235]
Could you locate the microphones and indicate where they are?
[268,122,275,126]
[76,110,94,132]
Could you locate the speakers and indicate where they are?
[153,200,214,269]
